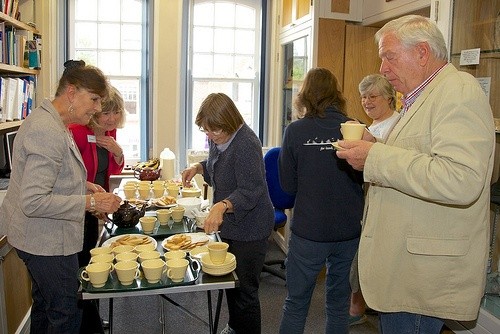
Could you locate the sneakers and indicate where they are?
[348,303,378,326]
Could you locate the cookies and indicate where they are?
[110,234,209,250]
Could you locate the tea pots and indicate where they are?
[105,200,148,229]
[133,167,162,181]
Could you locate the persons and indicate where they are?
[1,59,125,333]
[342,72,401,327]
[278,66,366,334]
[68,83,127,265]
[334,14,498,334]
[180,93,276,334]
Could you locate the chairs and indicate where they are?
[257,147,295,288]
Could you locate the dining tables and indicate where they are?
[81,177,241,334]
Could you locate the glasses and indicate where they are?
[360,94,383,102]
[199,126,223,136]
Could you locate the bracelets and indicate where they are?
[219,200,229,215]
[87,194,97,215]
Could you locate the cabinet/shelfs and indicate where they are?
[0,0,47,334]
[274,0,320,149]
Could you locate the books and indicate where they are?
[0,0,43,125]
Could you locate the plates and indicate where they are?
[120,200,151,208]
[162,233,212,253]
[101,234,158,253]
[155,201,177,208]
[201,252,236,276]
[190,247,209,258]
[332,142,345,150]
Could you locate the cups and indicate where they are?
[115,252,138,262]
[135,245,157,254]
[124,179,178,198]
[170,207,186,222]
[164,251,187,261]
[341,122,366,141]
[139,217,158,233]
[141,259,167,283]
[81,263,111,288]
[138,251,161,262]
[207,241,229,263]
[90,247,113,255]
[89,255,114,272]
[166,259,189,282]
[156,209,172,225]
[113,245,134,254]
[114,261,140,285]
[195,212,210,228]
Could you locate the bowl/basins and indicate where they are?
[181,188,201,197]
[177,197,202,216]
